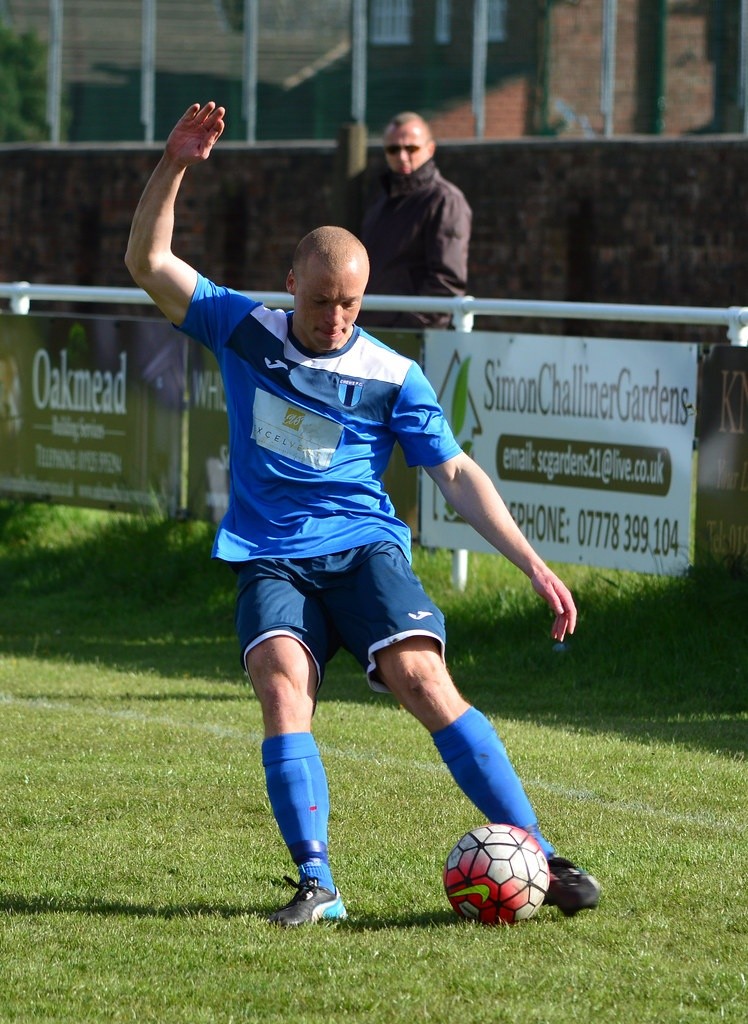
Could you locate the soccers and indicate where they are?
[442,823,550,926]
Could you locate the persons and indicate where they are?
[124,102,605,927]
[357,109,472,329]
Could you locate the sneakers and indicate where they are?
[269,876,348,928]
[540,858,600,916]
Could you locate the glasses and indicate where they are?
[384,144,428,154]
